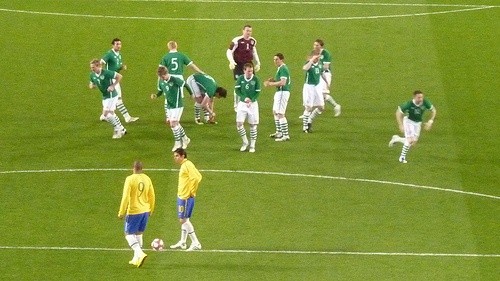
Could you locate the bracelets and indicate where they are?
[428,120,433,123]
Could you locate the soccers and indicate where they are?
[151,238,164,251]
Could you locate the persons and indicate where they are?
[159,41,205,124]
[100,38,139,123]
[89,59,126,139]
[151,65,195,152]
[118,162,155,268]
[187,73,227,125]
[170,148,202,250]
[226,25,260,112]
[299,39,341,133]
[234,63,259,152]
[264,53,291,142]
[389,91,436,163]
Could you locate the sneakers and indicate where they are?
[136,253,147,268]
[129,259,137,265]
[186,242,201,251]
[170,241,186,249]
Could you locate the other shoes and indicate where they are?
[112,127,126,139]
[195,118,204,125]
[299,114,303,119]
[303,123,313,134]
[249,145,255,153]
[270,132,282,138]
[334,105,341,116]
[240,142,249,152]
[125,116,139,123]
[399,157,407,163]
[205,118,218,125]
[277,135,290,142]
[182,138,190,149]
[388,135,397,148]
[100,117,109,122]
[171,142,182,151]
